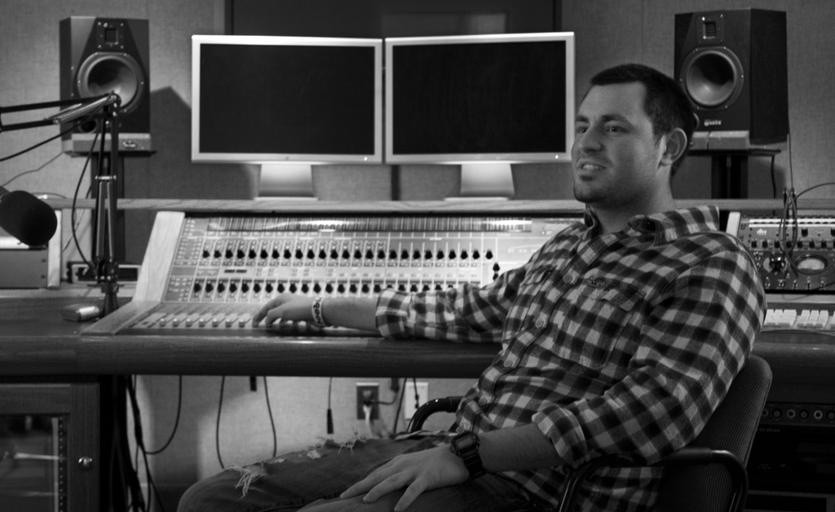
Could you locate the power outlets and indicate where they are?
[355,382,382,423]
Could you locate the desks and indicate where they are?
[0,286,835,512]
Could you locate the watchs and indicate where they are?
[451,428,488,481]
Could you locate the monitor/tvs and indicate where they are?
[384,31,575,200]
[191,35,383,202]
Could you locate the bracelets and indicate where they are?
[312,293,327,329]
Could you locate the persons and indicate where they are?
[172,63,774,512]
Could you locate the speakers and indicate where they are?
[673,8,788,150]
[59,17,151,152]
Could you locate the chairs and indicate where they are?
[405,352,775,512]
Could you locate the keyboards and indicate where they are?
[761,303,835,336]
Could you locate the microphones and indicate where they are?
[0,185,57,245]
